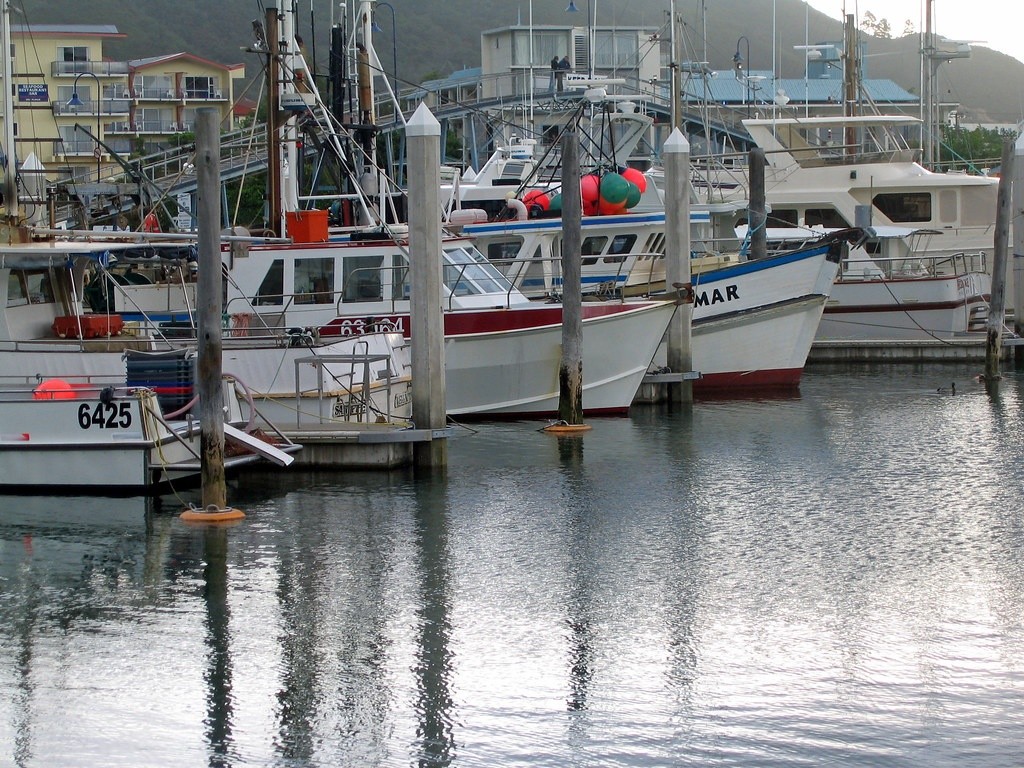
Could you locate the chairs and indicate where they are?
[134,89,144,98]
[182,123,190,132]
[214,90,222,99]
[169,122,178,132]
[122,122,130,131]
[181,89,188,98]
[167,88,175,98]
[135,122,141,131]
[123,89,129,98]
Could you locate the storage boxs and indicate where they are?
[286,210,328,243]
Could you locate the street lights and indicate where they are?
[65,69,103,183]
[732,35,754,120]
[565,0,593,80]
[371,1,398,124]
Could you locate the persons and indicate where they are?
[548,56,573,91]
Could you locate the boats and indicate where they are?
[1,1,1024,486]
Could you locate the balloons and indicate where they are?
[523,189,561,212]
[580,168,647,214]
